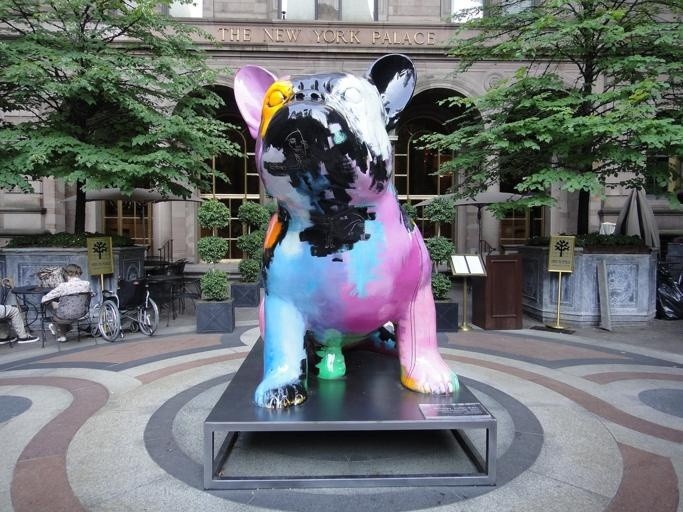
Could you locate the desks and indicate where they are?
[11,285,59,341]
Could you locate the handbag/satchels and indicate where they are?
[37,265,66,289]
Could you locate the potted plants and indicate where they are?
[196,199,235,333]
[426,199,459,333]
[231,201,267,306]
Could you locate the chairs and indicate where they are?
[40,293,92,353]
[145,239,189,325]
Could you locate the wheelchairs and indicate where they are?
[97,278,159,342]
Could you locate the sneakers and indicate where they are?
[0,337,18,345]
[17,334,40,344]
[49,323,56,336]
[56,337,66,342]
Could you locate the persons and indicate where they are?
[41,262,97,343]
[0,304,40,346]
